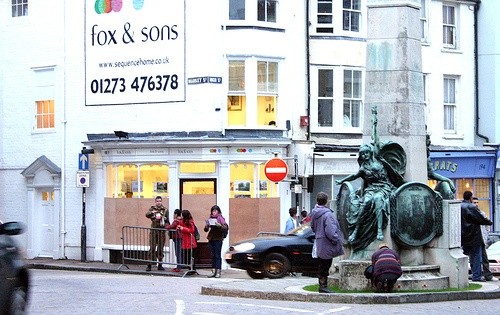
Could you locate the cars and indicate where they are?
[0,221,31,315]
[225,222,320,279]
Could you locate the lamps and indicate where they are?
[115,131,128,139]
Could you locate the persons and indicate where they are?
[371,245,403,293]
[145,196,170,271]
[311,192,346,293]
[336,106,397,241]
[203,205,228,278]
[300,211,312,225]
[285,206,301,234]
[164,209,197,272]
[461,191,499,282]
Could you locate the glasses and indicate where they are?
[473,201,479,204]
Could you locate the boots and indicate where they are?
[207,268,222,279]
[318,276,335,294]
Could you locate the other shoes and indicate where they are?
[172,268,183,273]
[375,282,383,293]
[146,264,151,271]
[488,276,500,281]
[158,264,165,271]
[468,270,475,280]
[472,276,486,282]
[387,286,394,293]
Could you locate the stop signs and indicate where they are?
[265,158,287,183]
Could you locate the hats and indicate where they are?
[379,242,390,249]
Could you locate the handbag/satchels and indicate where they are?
[364,264,374,279]
[193,224,200,241]
[312,238,319,260]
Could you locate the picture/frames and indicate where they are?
[230,96,242,111]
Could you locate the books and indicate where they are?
[206,218,216,226]
